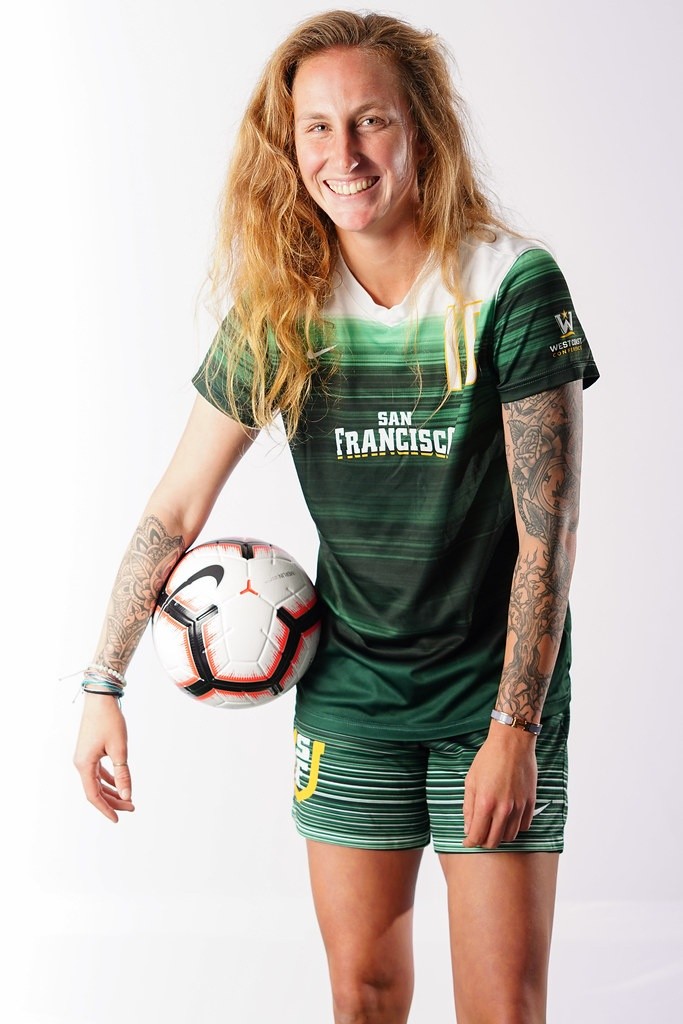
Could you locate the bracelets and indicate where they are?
[58,662,128,710]
[491,708,543,735]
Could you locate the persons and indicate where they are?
[74,10,602,1023]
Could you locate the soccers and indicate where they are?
[151,537,320,707]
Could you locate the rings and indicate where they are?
[112,763,129,767]
